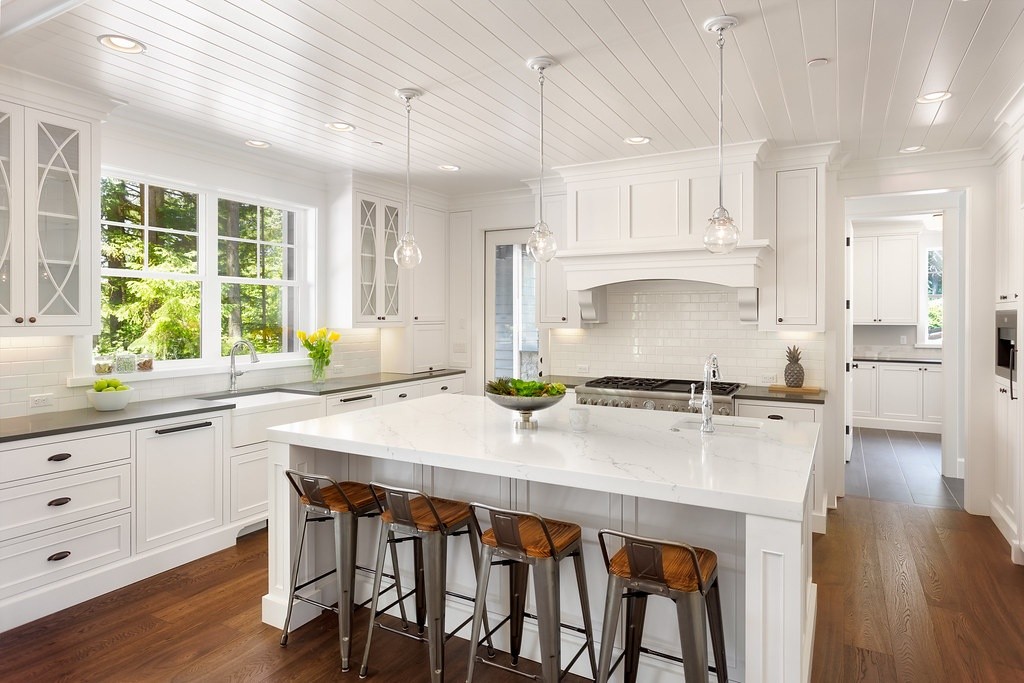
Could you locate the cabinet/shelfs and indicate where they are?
[379,201,449,374]
[0,409,233,633]
[852,359,942,435]
[989,381,1023,546]
[992,148,1024,304]
[229,440,270,549]
[732,396,829,534]
[851,234,917,326]
[323,188,408,328]
[1,93,103,339]
[326,372,468,417]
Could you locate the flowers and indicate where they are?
[297,325,343,382]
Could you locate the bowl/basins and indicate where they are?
[86,387,135,412]
[484,391,566,430]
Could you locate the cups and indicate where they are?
[568,407,591,433]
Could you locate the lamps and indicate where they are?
[392,87,424,268]
[702,14,743,258]
[525,55,559,263]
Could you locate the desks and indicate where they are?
[260,391,822,683]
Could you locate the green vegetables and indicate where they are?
[484,375,566,397]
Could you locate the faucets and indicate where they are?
[700,352,723,433]
[228,339,261,393]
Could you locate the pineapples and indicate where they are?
[783,345,805,388]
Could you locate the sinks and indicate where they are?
[194,387,320,410]
[670,415,763,438]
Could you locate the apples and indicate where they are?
[90,377,129,392]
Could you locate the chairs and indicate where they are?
[279,468,410,673]
[595,527,730,683]
[464,498,599,683]
[358,479,499,683]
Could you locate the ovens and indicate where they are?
[993,309,1018,382]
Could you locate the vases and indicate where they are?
[309,355,327,384]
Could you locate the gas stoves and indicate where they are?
[574,375,747,417]
